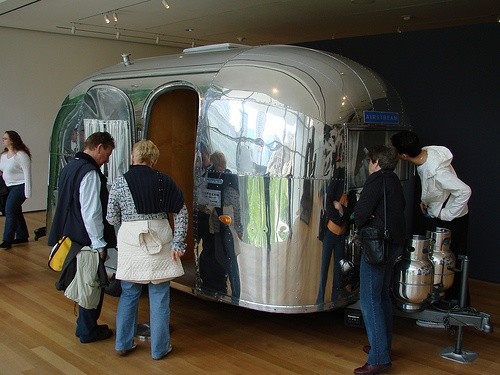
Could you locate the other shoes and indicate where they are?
[0,241,12,249]
[168,344,173,352]
[117,343,137,356]
[11,238,29,246]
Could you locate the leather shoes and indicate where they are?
[75,324,112,343]
[363,345,371,353]
[354,363,392,375]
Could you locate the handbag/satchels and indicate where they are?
[360,227,384,265]
[48,235,72,272]
[319,215,328,241]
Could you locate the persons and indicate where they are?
[0,130,33,249]
[196,152,243,303]
[47,131,117,344]
[345,147,406,375]
[391,130,474,328]
[287,165,293,239]
[105,138,190,361]
[263,171,272,242]
[197,142,212,207]
[316,166,358,309]
[66,127,80,160]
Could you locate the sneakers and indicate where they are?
[416,320,445,328]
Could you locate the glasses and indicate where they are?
[366,159,372,163]
